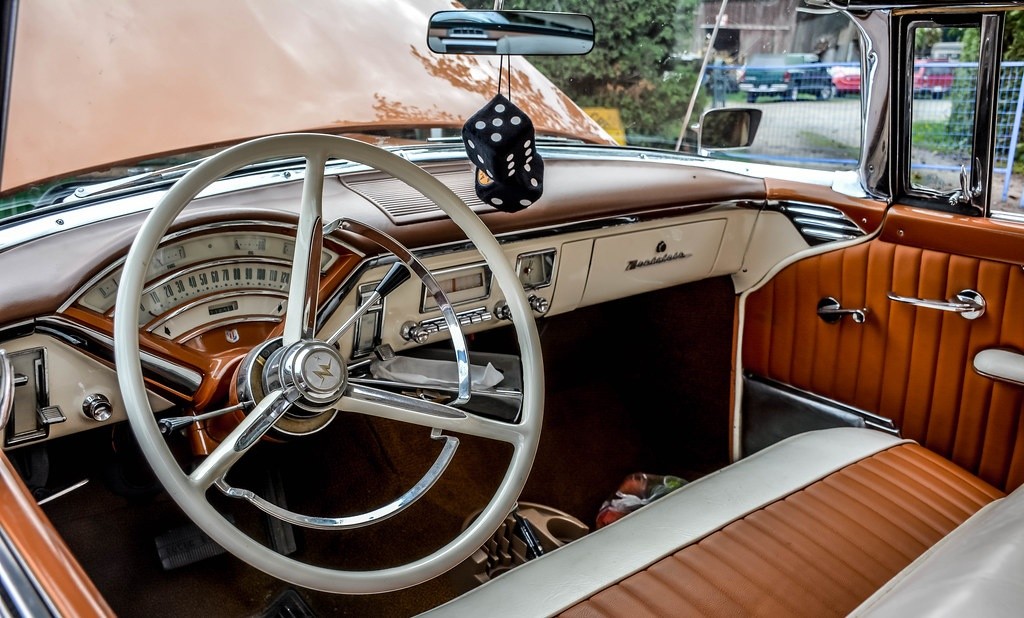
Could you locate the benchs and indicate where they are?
[413,424,1024,618]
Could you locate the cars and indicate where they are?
[664,52,741,96]
[738,52,861,102]
[913,58,954,99]
